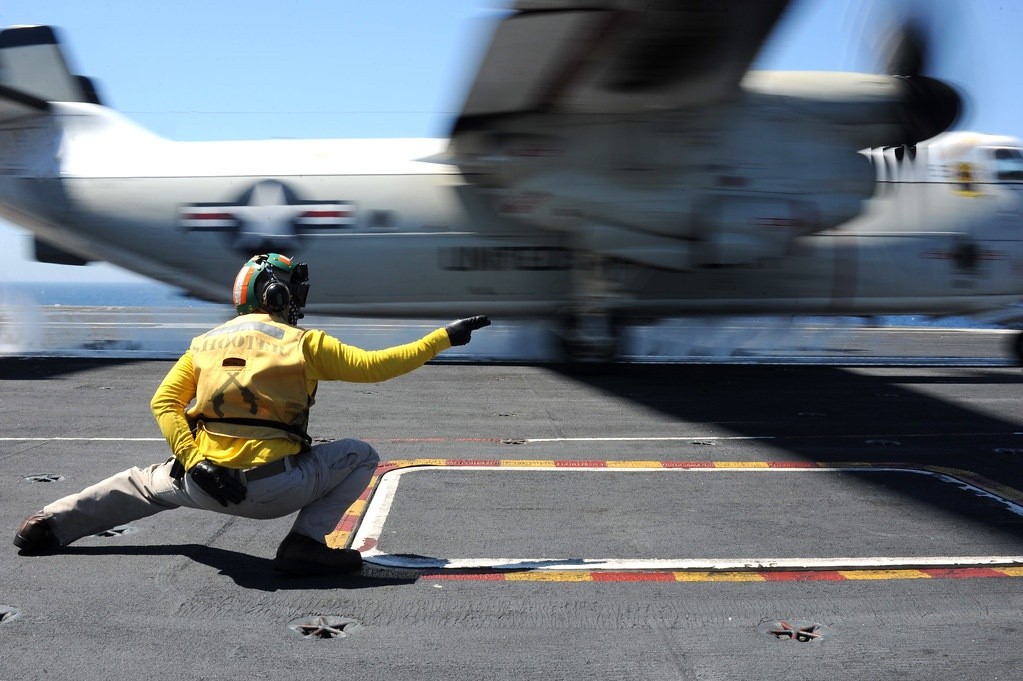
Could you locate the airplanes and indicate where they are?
[1,0,1022,369]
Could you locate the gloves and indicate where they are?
[445,314,491,345]
[190,460,247,507]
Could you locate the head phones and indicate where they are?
[250,254,290,312]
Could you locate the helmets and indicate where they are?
[233,252,311,318]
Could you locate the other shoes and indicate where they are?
[12,508,56,551]
[275,530,364,570]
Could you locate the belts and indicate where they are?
[230,454,297,481]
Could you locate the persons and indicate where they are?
[13,253,493,583]
